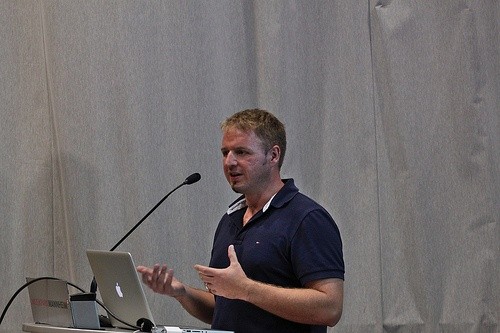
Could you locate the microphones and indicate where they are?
[90,173,201,293]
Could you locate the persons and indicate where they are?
[136,108,345,333]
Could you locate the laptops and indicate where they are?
[85,249,235,333]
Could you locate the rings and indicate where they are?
[205,283,209,290]
